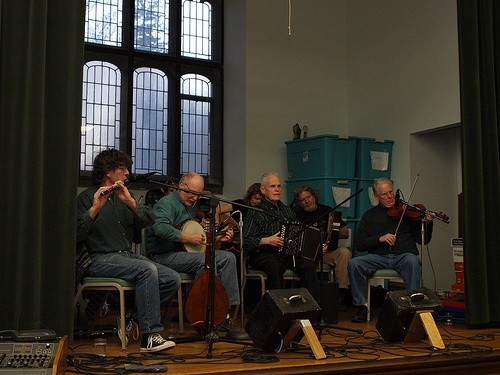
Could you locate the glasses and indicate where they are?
[185,183,202,198]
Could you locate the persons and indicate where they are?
[242,171,339,326]
[144,171,249,341]
[347,178,436,323]
[293,184,354,314]
[210,182,264,284]
[76,149,180,353]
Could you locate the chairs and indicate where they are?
[70,240,424,350]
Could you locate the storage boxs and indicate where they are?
[284,133,395,253]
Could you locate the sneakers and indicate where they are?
[140,333,177,352]
[117,310,139,347]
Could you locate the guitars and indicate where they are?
[186,221,229,334]
[179,219,240,253]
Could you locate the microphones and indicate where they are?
[128,172,157,182]
[395,189,400,209]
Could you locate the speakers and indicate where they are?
[245,287,322,352]
[376,285,444,343]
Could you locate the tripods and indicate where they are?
[289,188,363,340]
[146,179,281,359]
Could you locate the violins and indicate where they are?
[389,199,450,223]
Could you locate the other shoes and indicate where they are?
[351,307,374,322]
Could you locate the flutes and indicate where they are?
[100,179,130,199]
